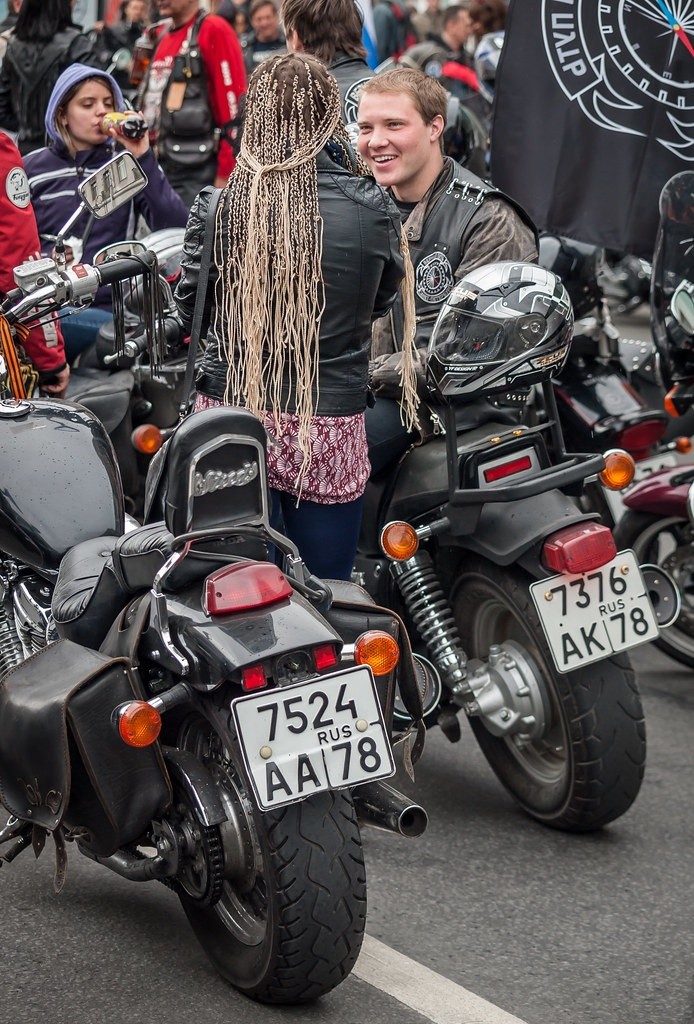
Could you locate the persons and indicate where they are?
[0,0,540,583]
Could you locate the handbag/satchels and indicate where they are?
[160,55,218,179]
[125,377,185,522]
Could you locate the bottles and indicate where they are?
[98,112,148,140]
[129,30,153,84]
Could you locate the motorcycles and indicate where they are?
[102,227,694,832]
[1,151,441,1009]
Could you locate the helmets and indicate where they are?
[425,261,575,403]
[130,227,188,282]
